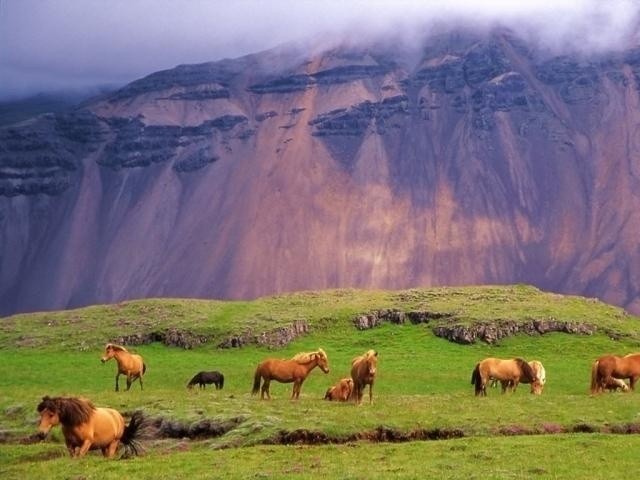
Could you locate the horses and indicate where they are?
[350,349,378,407]
[591,353,639,393]
[100,344,146,391]
[36,395,150,459]
[324,378,354,402]
[471,356,546,397]
[186,371,224,391]
[250,348,330,400]
